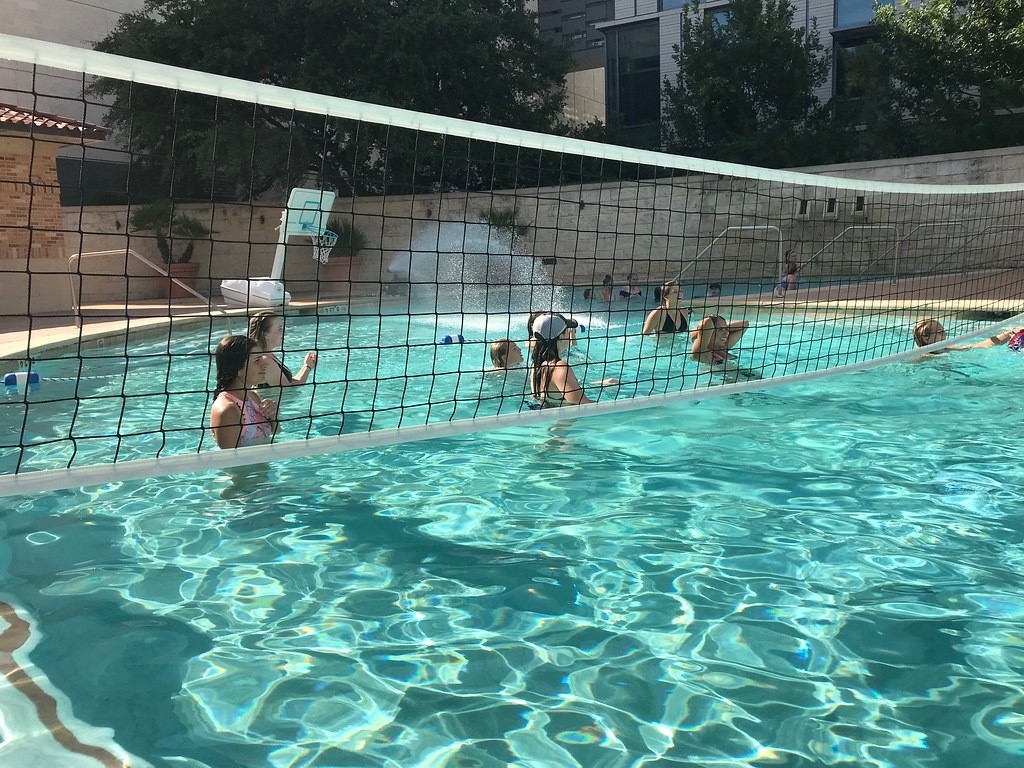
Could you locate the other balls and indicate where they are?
[1006,326,1024,349]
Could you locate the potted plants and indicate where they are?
[130,197,220,298]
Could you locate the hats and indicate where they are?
[532,314,578,341]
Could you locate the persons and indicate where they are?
[491,338,524,368]
[624,273,641,298]
[583,288,594,299]
[709,282,720,295]
[599,275,615,302]
[776,250,807,291]
[528,311,619,404]
[248,310,316,388]
[643,281,689,333]
[912,319,1024,358]
[690,313,749,363]
[211,335,280,448]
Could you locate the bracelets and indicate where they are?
[306,366,311,371]
